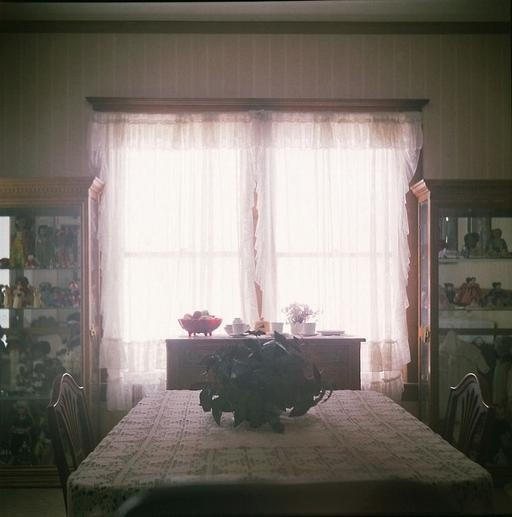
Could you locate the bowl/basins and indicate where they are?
[224,324,251,336]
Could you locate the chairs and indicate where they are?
[432,370,494,466]
[47,368,100,517]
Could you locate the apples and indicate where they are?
[185,310,215,320]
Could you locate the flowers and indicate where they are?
[283,302,318,323]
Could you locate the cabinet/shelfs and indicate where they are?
[409,177,512,477]
[0,175,107,487]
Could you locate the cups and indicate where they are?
[303,322,316,334]
[271,322,284,333]
[232,318,243,336]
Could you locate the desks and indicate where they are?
[67,386,494,514]
[164,333,366,391]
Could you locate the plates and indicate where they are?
[267,332,288,338]
[300,333,319,336]
[317,330,345,336]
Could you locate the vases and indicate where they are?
[290,321,316,334]
